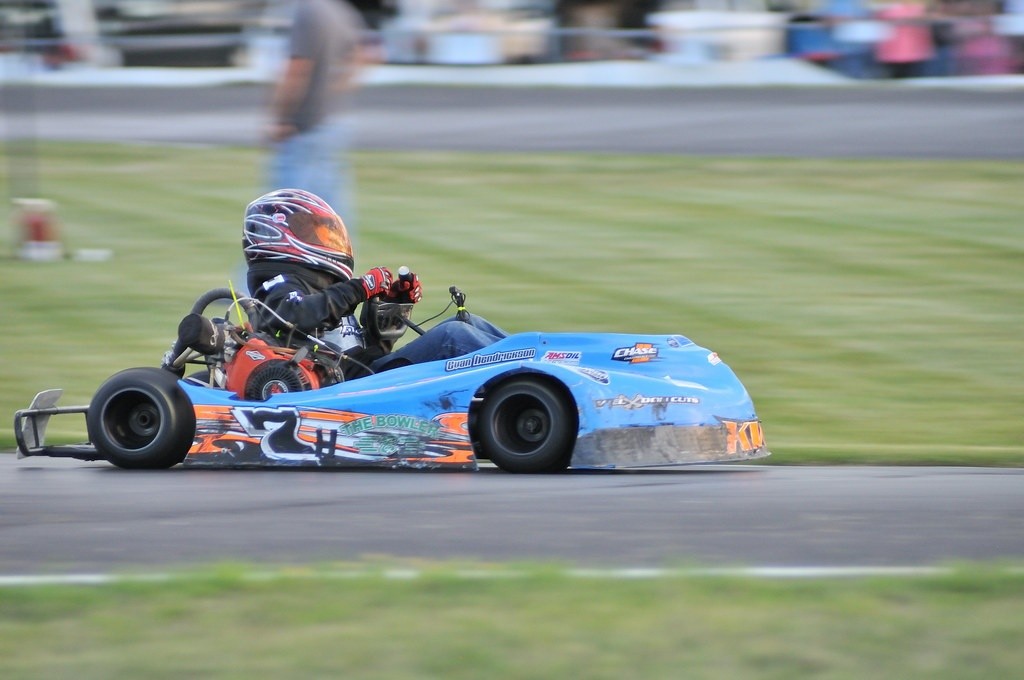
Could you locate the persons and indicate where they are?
[262,0,365,247]
[242,186,511,393]
[871,0,1022,81]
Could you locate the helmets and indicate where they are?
[242,188,354,282]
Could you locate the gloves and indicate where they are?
[394,272,423,304]
[362,266,394,299]
